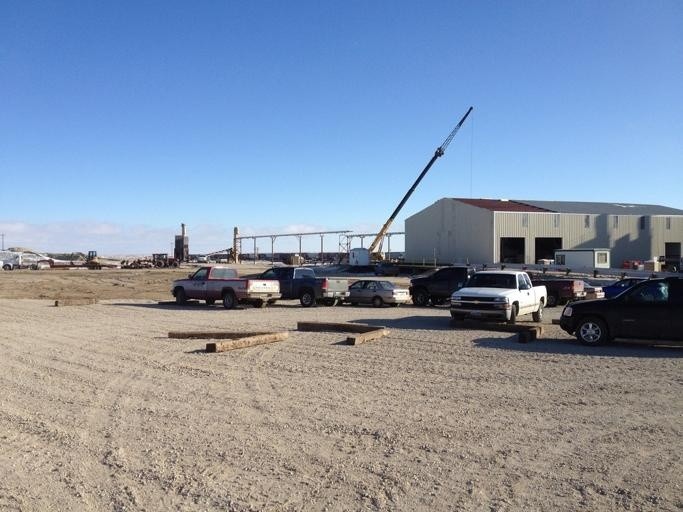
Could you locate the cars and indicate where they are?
[559,275,682,347]
[338,279,411,308]
[602,276,668,300]
[582,281,605,300]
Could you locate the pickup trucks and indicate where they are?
[449,270,548,326]
[257,266,352,308]
[170,265,284,310]
[216,257,228,263]
[527,272,584,307]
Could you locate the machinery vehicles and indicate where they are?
[129,253,180,269]
[85,251,102,270]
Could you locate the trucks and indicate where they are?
[3,255,38,270]
[408,266,476,307]
